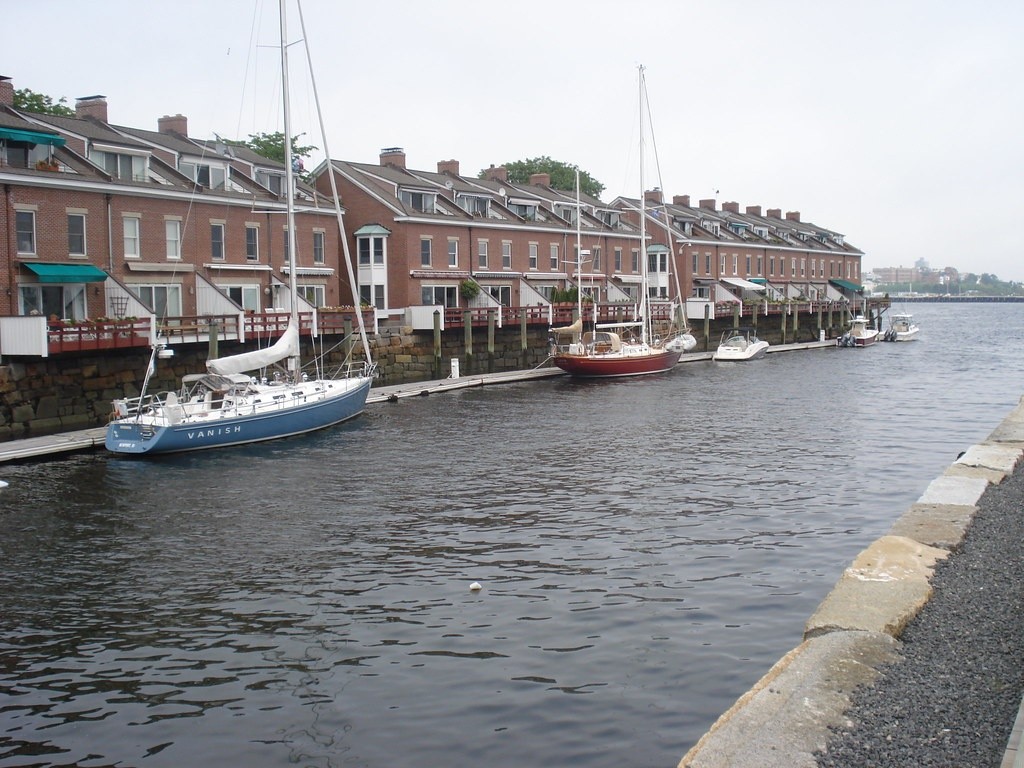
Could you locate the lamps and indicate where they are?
[158,345,174,359]
[95,287,100,295]
[189,286,194,294]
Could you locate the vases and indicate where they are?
[50,315,56,320]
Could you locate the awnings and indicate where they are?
[23,263,109,283]
[721,278,765,291]
[0,127,66,147]
[828,279,865,291]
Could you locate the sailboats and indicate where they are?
[545,64,697,377]
[103,0,378,456]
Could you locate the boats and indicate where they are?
[878,312,920,342]
[713,327,770,361]
[835,317,880,346]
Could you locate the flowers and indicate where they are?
[65,316,137,326]
[321,302,370,310]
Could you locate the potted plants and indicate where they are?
[36,160,59,171]
